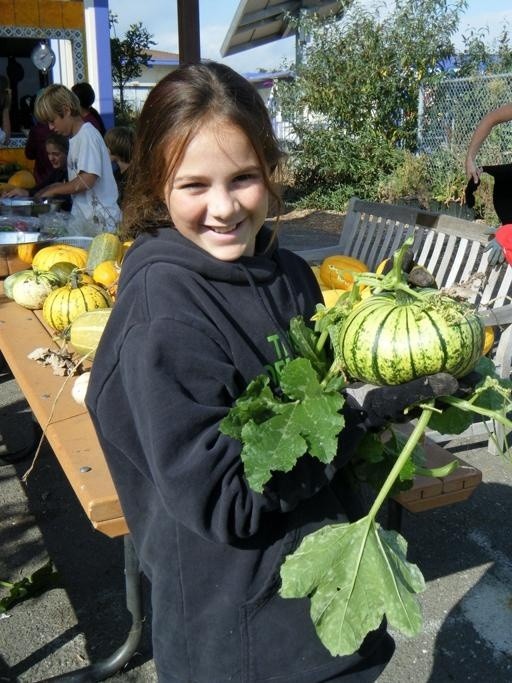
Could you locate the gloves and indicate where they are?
[375,248,438,300]
[336,354,499,434]
[480,237,506,266]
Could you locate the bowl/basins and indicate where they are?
[8,137,28,146]
[1,197,68,217]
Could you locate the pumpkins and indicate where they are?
[8,171,35,189]
[3,233,134,362]
[319,256,494,385]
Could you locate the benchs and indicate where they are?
[290,192,512,459]
[378,415,485,542]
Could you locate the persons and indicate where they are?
[0,87,12,146]
[31,80,123,231]
[1,133,70,203]
[86,58,488,681]
[463,100,511,184]
[26,120,67,186]
[103,126,136,181]
[72,81,103,135]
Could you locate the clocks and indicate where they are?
[29,39,56,75]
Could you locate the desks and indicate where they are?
[0,269,370,678]
